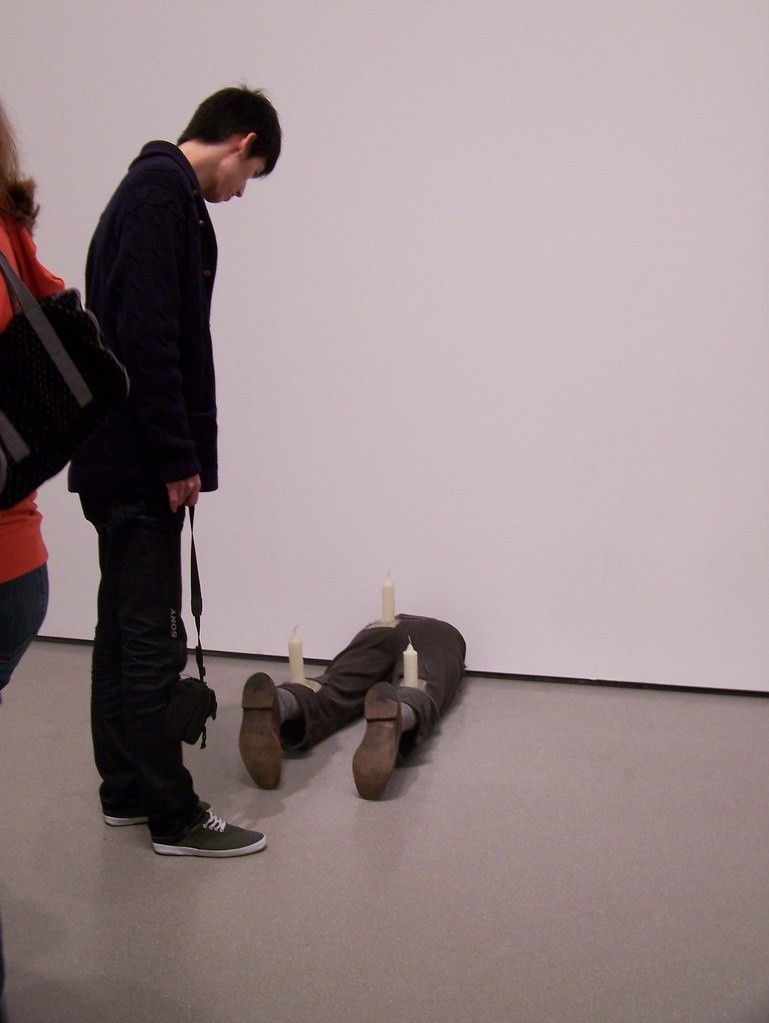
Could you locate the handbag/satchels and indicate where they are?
[182,678,217,750]
[0,288,129,510]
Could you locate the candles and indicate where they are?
[284,627,322,695]
[398,634,428,693]
[367,571,402,630]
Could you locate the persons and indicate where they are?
[67,86,283,857]
[239,613,466,801]
[0,105,64,690]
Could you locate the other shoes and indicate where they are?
[237,672,284,788]
[351,682,404,801]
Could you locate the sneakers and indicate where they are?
[151,812,267,857]
[103,801,213,825]
